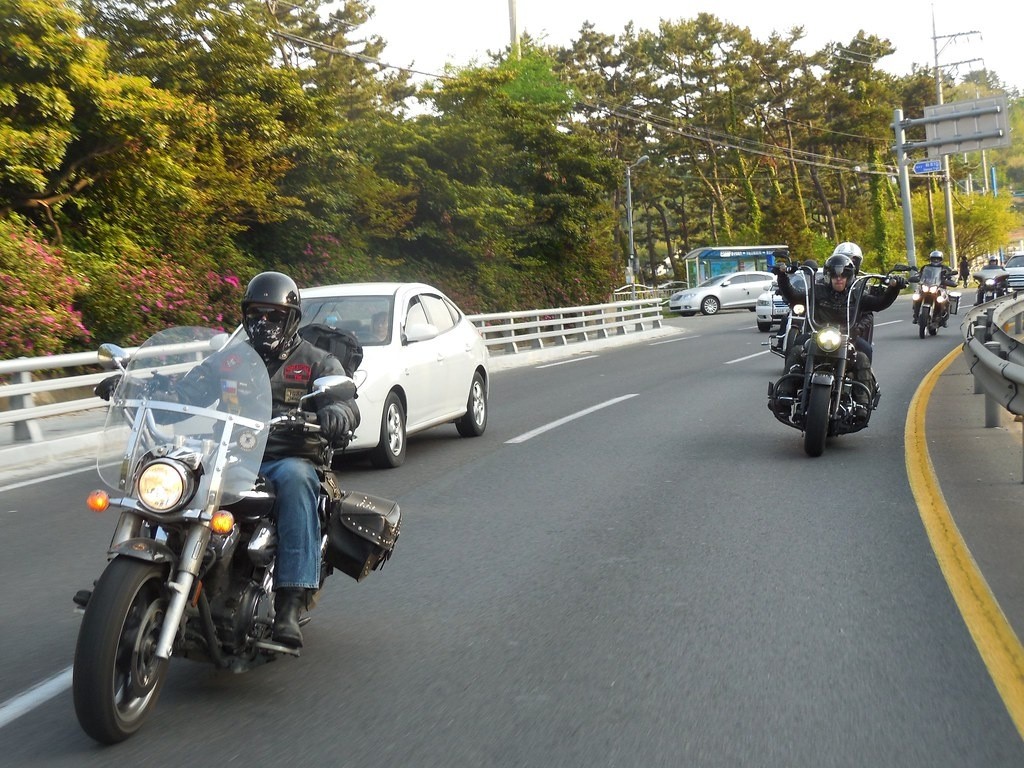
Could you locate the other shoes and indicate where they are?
[852,408,868,424]
[913,318,917,324]
[974,301,982,306]
[768,399,791,415]
[943,320,948,327]
[963,284,967,287]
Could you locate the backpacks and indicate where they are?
[298,323,363,399]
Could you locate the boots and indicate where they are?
[771,261,788,275]
[272,589,309,647]
[74,589,93,606]
[890,276,909,290]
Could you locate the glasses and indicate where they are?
[829,271,852,278]
[244,306,288,326]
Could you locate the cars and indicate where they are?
[209,281,492,471]
[655,280,688,289]
[756,267,825,332]
[612,284,654,295]
[669,271,777,317]
[1004,254,1024,295]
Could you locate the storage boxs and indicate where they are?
[327,490,402,583]
[949,291,962,313]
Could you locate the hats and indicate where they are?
[371,311,387,320]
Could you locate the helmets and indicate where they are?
[989,257,998,265]
[833,242,864,266]
[929,251,944,266]
[803,261,818,269]
[240,272,301,322]
[823,255,855,287]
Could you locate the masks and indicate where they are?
[248,320,286,353]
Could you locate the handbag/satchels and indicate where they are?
[326,490,402,582]
[959,275,962,280]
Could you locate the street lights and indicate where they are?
[627,155,649,267]
[912,160,941,174]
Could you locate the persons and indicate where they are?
[974,256,1010,306]
[958,256,970,288]
[908,250,958,328]
[94,271,362,658]
[351,312,389,342]
[772,242,909,420]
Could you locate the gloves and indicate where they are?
[315,402,351,445]
[93,375,146,401]
[909,276,915,282]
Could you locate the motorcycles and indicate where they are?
[908,265,962,339]
[73,325,403,745]
[972,268,1013,302]
[765,247,913,457]
